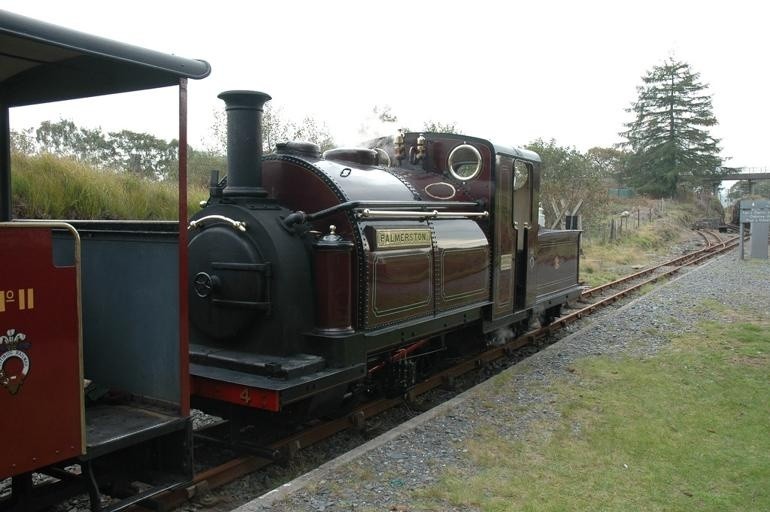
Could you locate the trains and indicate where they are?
[0,8,585,511]
